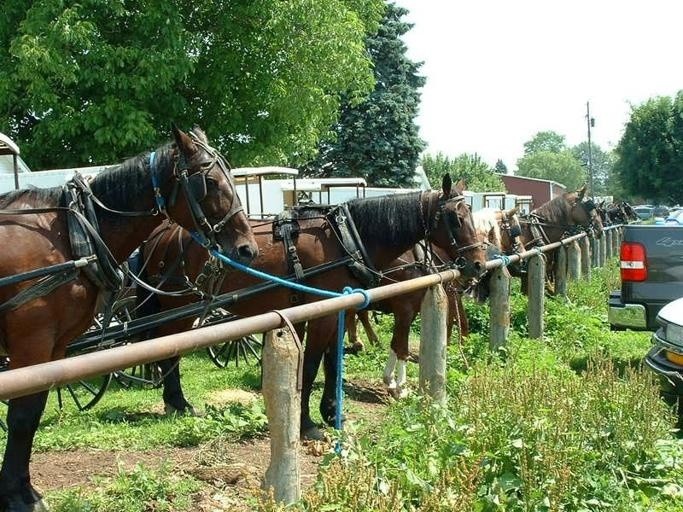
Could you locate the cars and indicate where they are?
[606,202,683,395]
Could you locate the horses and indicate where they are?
[351,207,524,399]
[0,120,260,512]
[135,172,488,445]
[526,188,638,310]
